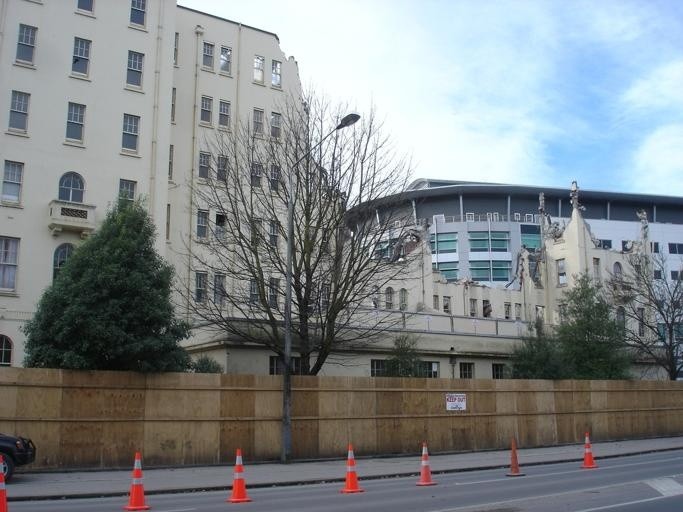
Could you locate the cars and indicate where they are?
[0,431,37,482]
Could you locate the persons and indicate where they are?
[514,244,529,286]
[634,208,649,244]
[567,183,586,210]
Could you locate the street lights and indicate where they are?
[277,111,361,463]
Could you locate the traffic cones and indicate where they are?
[576,430,600,469]
[223,446,255,504]
[502,437,530,477]
[0,454,9,512]
[335,443,367,495]
[120,450,153,512]
[413,441,441,489]
